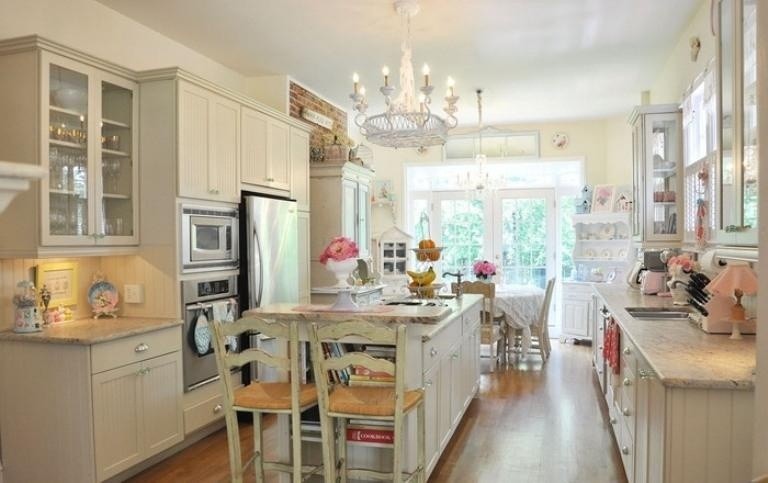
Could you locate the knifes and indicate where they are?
[683,271,712,318]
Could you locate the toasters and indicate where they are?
[640,268,669,294]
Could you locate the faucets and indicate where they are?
[670,279,689,291]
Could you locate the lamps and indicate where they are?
[348,0,484,154]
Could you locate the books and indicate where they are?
[321,342,396,443]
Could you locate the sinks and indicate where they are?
[628,313,692,319]
[627,305,699,314]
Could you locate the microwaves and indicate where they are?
[179,202,239,273]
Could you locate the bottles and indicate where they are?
[44,305,74,324]
[47,120,126,238]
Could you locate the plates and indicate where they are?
[571,222,629,282]
[87,280,120,311]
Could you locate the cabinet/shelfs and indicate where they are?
[279,301,482,483]
[291,127,311,212]
[627,101,683,250]
[137,82,293,206]
[709,0,768,262]
[372,225,414,280]
[0,326,182,482]
[559,212,639,343]
[310,160,376,301]
[183,384,245,436]
[591,295,756,483]
[0,50,141,247]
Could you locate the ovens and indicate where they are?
[181,271,244,393]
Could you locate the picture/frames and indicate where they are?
[33,261,79,310]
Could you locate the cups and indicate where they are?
[653,190,676,202]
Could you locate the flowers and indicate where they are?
[472,260,497,279]
[319,236,360,267]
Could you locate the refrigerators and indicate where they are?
[239,193,298,383]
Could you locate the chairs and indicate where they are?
[453,275,557,368]
[206,315,428,483]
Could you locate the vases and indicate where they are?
[324,257,359,292]
